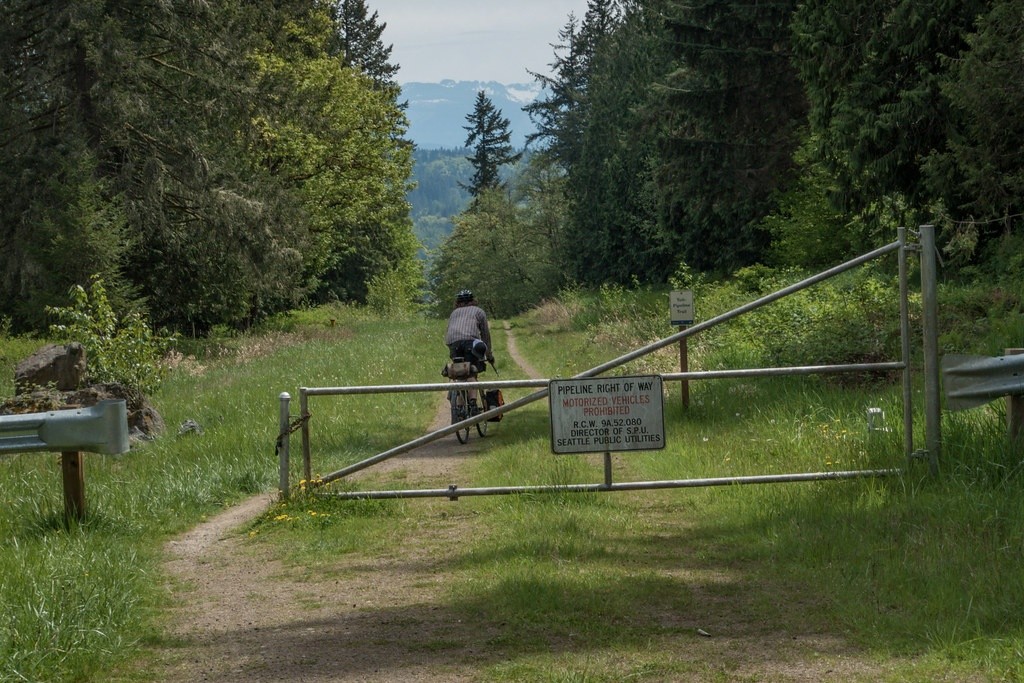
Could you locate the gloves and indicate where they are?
[487,356,495,364]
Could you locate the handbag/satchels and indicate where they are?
[447,362,470,378]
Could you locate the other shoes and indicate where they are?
[470,398,480,415]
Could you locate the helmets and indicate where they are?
[457,290,473,303]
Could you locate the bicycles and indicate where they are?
[450,357,493,444]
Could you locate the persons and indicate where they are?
[446,289,494,408]
